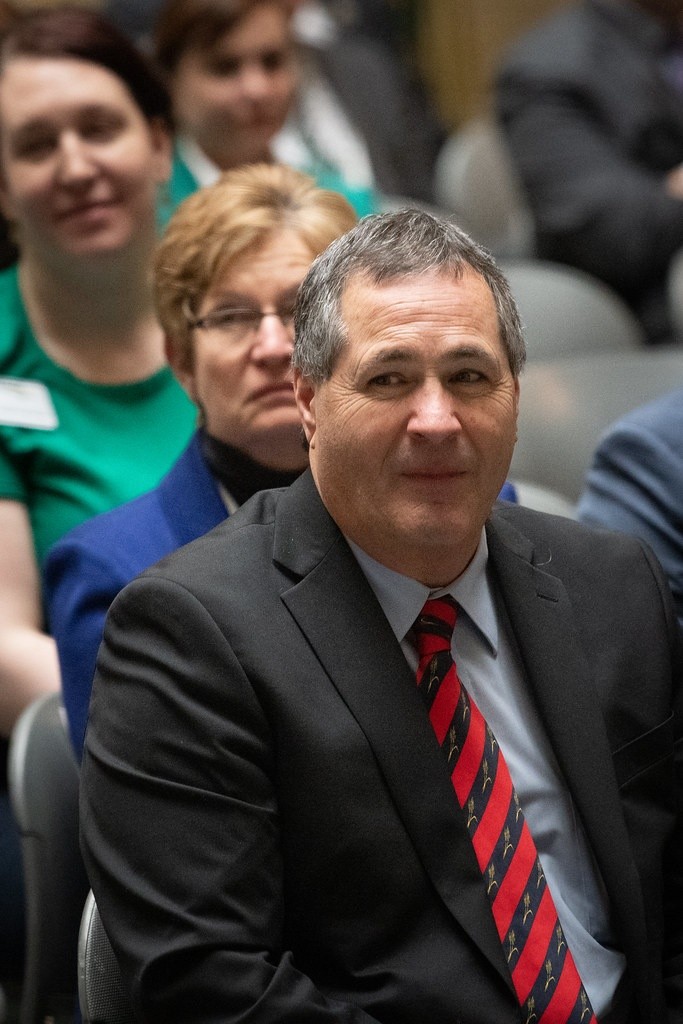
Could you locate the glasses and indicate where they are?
[175,302,296,344]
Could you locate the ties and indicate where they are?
[399,599,599,1023]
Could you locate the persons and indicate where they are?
[77,206,683,1024]
[574,383,683,687]
[483,0,681,351]
[143,0,380,316]
[266,0,461,219]
[3,4,284,1020]
[38,160,526,898]
